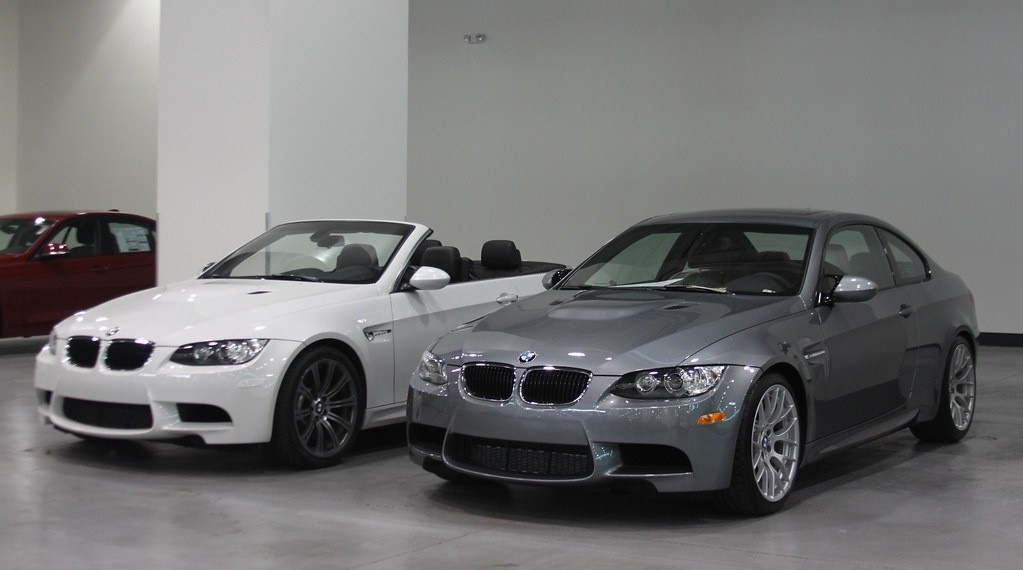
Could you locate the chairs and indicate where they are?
[338,244,381,279]
[68,223,95,256]
[680,236,741,289]
[420,245,460,285]
[822,244,846,293]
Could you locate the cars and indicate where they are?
[404,208,981,514]
[1,208,159,339]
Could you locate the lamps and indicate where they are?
[463,31,486,45]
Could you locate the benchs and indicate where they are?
[411,240,522,279]
[760,250,880,289]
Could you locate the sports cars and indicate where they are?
[31,218,573,469]
